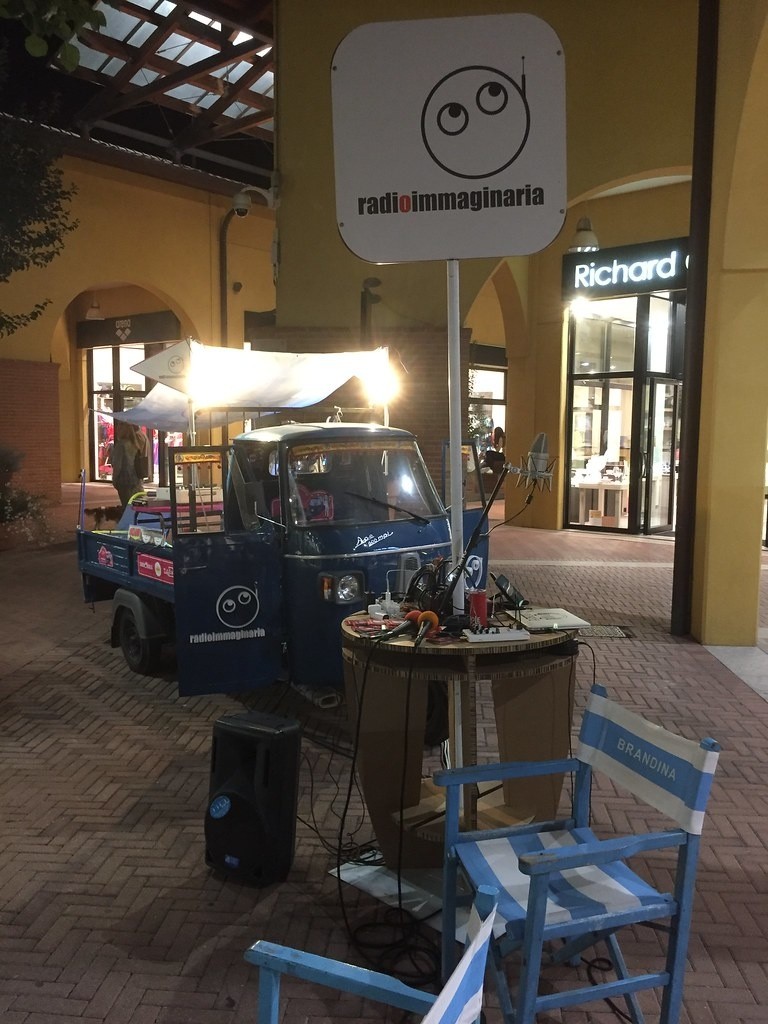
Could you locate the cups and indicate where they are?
[470,590,487,628]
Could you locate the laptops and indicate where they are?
[505,607,591,632]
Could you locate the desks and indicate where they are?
[577,482,628,527]
[129,503,224,538]
[341,601,579,871]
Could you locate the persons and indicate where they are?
[112,419,147,505]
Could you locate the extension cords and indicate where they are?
[375,598,400,615]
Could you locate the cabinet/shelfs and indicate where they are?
[662,406,679,480]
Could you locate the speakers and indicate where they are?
[202,712,301,886]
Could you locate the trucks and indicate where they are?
[76,398,490,747]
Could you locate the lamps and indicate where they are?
[85,291,105,320]
[567,202,599,252]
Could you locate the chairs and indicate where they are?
[269,482,335,522]
[243,885,501,1024]
[433,681,724,1024]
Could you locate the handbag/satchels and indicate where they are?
[134,456,149,479]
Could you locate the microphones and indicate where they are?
[414,610,439,649]
[380,610,423,641]
[523,432,550,492]
